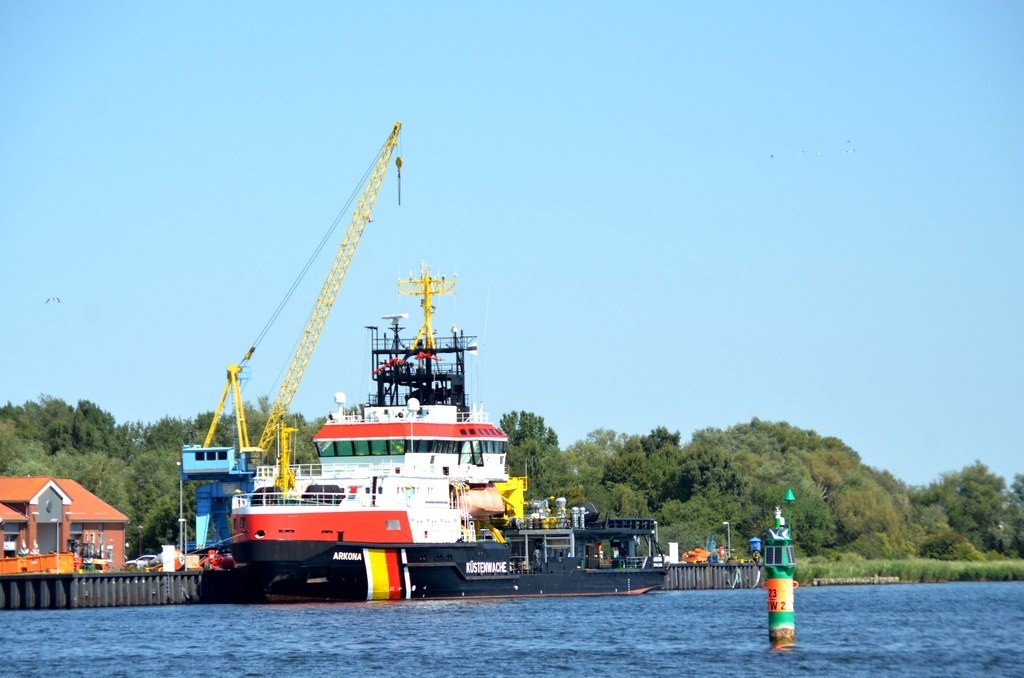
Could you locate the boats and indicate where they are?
[231,258,673,603]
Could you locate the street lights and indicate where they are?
[50,518,59,574]
[178,518,188,572]
[722,520,731,558]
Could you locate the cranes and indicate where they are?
[180,120,406,486]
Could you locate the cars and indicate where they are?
[124,555,158,570]
